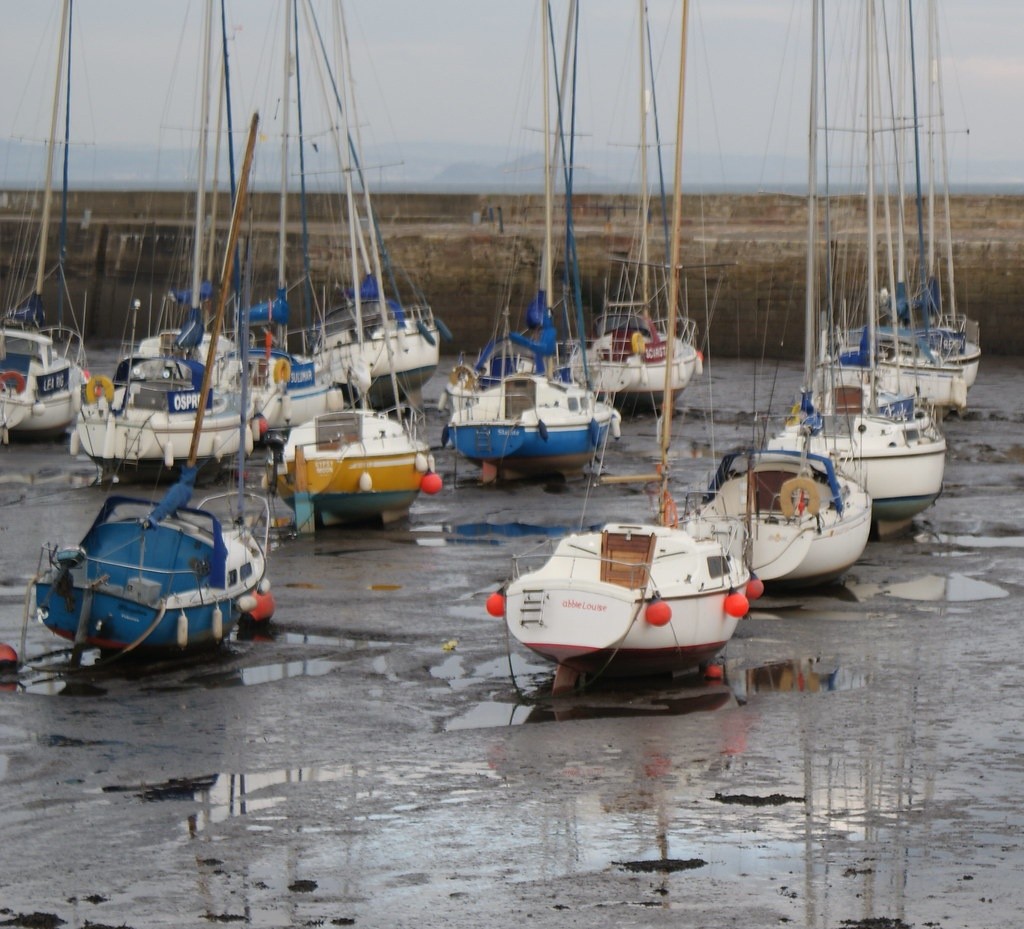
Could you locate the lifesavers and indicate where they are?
[274,358,291,383]
[450,365,475,389]
[779,476,821,517]
[0,370,25,394]
[86,375,114,403]
[792,403,801,422]
[631,333,645,352]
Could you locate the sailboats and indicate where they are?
[2,2,980,575]
[485,0,768,691]
[1,110,283,690]
